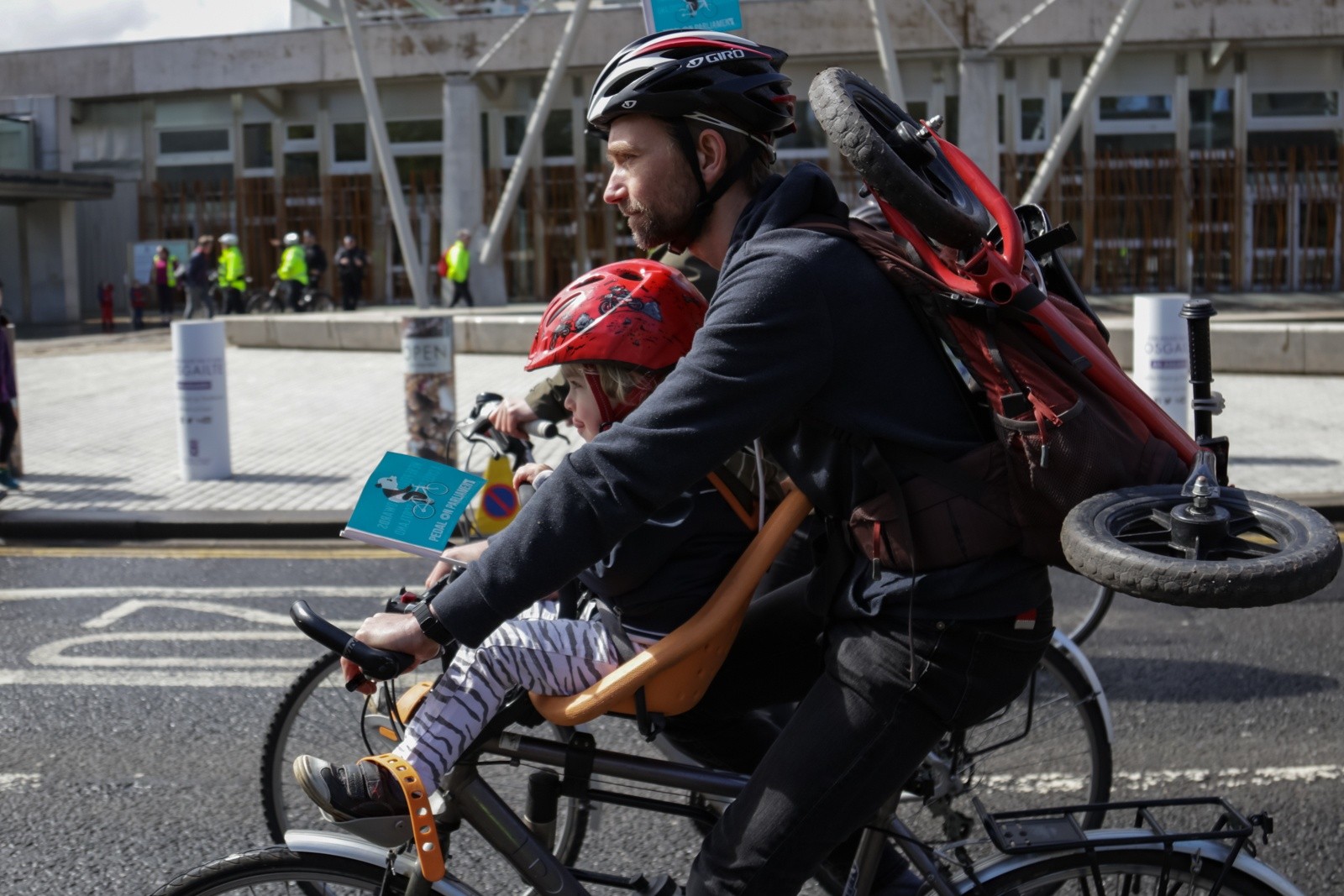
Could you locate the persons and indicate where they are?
[294,256,781,848]
[341,29,1052,895]
[129,280,145,327]
[183,236,214,318]
[445,232,474,307]
[98,280,114,323]
[150,246,178,325]
[217,233,247,314]
[270,230,327,312]
[334,236,365,311]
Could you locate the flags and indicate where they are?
[349,451,488,551]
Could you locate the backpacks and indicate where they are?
[740,211,1133,509]
[438,243,454,278]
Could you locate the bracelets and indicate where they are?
[411,595,453,645]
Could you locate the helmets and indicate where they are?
[282,232,298,245]
[587,29,798,165]
[217,233,239,246]
[525,260,709,372]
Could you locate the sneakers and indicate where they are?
[292,755,412,847]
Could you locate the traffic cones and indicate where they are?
[462,453,525,541]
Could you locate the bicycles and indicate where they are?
[241,271,337,313]
[140,389,1314,895]
[803,64,1343,612]
